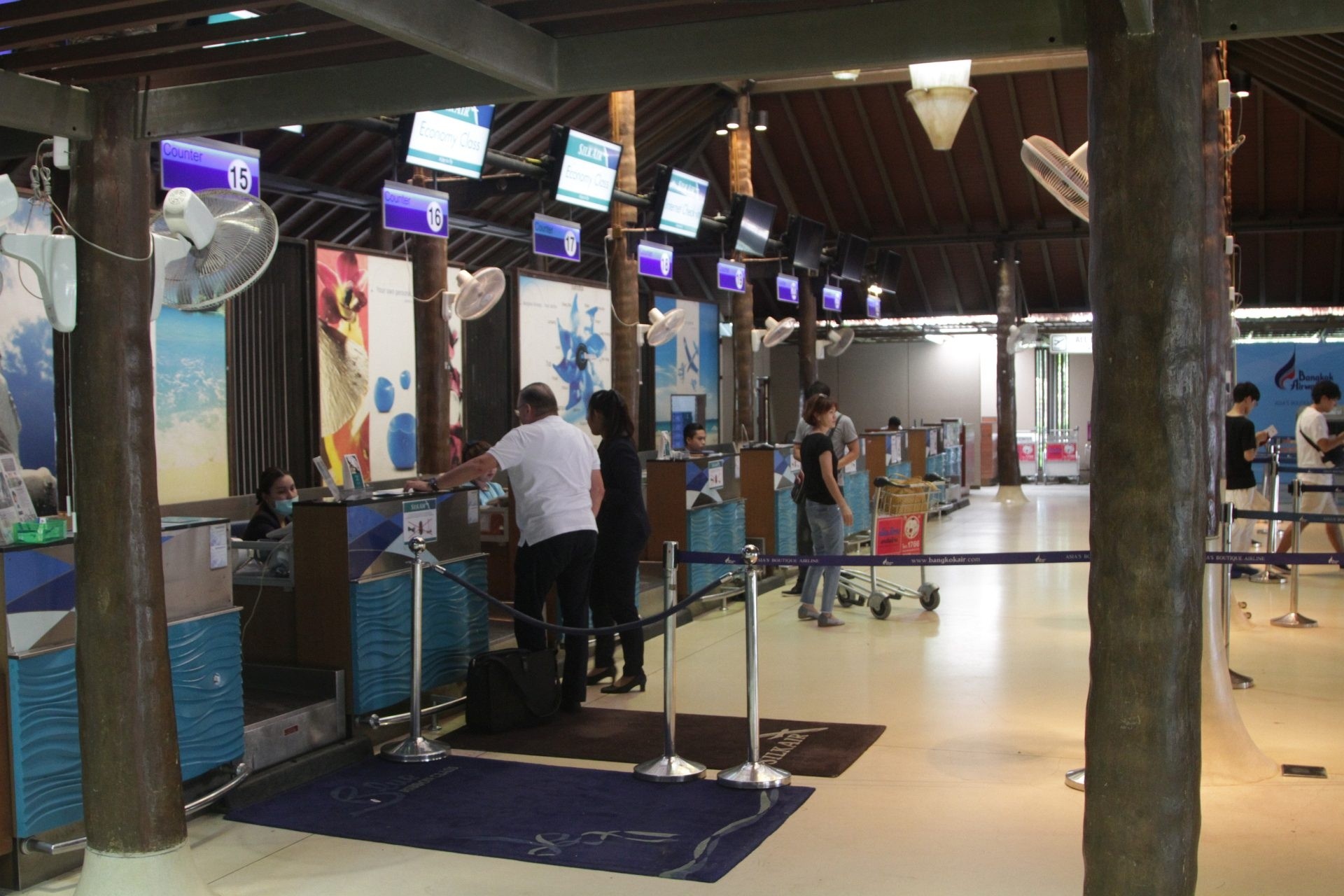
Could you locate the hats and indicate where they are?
[888,416,901,428]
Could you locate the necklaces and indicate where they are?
[478,485,493,500]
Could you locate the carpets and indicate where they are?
[223,753,817,883]
[433,707,886,778]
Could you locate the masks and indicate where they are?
[274,495,299,515]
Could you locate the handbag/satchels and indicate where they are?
[880,474,937,513]
[1319,448,1342,468]
[791,484,807,504]
[467,647,561,731]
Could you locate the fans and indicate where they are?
[815,328,855,360]
[636,308,687,347]
[147,187,279,394]
[442,267,506,322]
[751,316,796,352]
[1021,135,1092,223]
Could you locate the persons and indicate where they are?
[797,395,854,626]
[1273,380,1344,571]
[889,416,901,430]
[243,466,298,541]
[683,422,706,454]
[585,390,650,692]
[782,380,859,596]
[461,440,506,505]
[1225,381,1270,578]
[404,381,605,711]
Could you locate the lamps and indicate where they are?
[904,59,977,150]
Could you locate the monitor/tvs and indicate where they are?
[788,214,828,272]
[837,233,869,284]
[653,165,711,241]
[548,125,624,214]
[876,249,903,294]
[398,104,497,183]
[730,194,777,258]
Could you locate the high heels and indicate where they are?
[586,664,616,686]
[601,671,646,694]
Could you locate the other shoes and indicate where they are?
[817,614,845,627]
[1231,564,1258,578]
[798,606,821,619]
[562,701,582,714]
[1340,564,1344,569]
[1271,563,1291,573]
[782,586,802,596]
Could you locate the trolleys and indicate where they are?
[822,472,950,620]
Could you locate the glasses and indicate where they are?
[513,404,523,417]
[818,393,828,398]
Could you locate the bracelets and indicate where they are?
[428,478,439,491]
